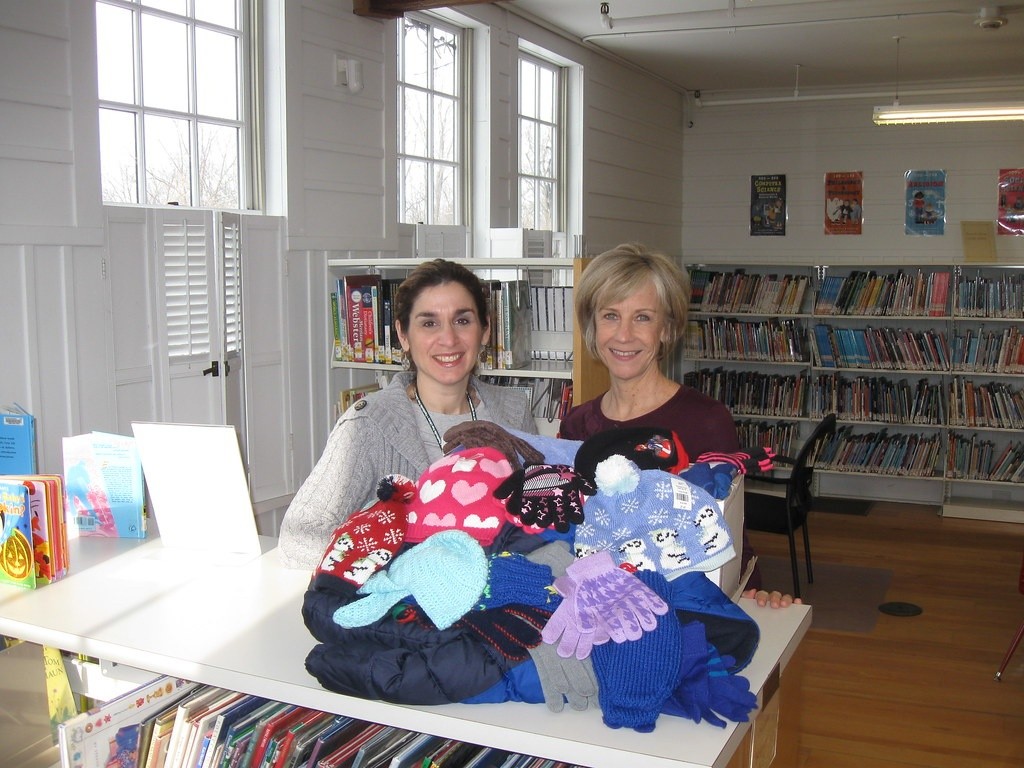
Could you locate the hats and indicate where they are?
[573,454,736,584]
[310,473,419,589]
[403,445,514,546]
[573,426,689,490]
[331,529,489,632]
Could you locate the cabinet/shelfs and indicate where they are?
[672,261,1024,524]
[327,257,611,441]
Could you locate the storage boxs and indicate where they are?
[687,462,758,604]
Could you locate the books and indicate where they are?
[959,272,1024,319]
[1,474,72,589]
[810,374,947,425]
[686,268,808,314]
[333,383,384,424]
[805,426,943,478]
[329,273,573,371]
[0,402,38,475]
[61,430,148,539]
[949,325,1024,375]
[684,366,808,418]
[735,420,800,468]
[814,270,952,318]
[479,375,573,439]
[683,318,808,362]
[947,377,1024,481]
[56,676,591,768]
[808,324,950,371]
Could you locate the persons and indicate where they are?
[557,243,804,609]
[276,259,539,567]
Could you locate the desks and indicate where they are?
[0,517,811,768]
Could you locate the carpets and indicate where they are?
[754,555,894,633]
[810,494,876,516]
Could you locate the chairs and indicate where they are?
[743,412,845,598]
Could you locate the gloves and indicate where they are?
[442,420,545,472]
[588,641,661,728]
[605,568,684,711]
[679,622,759,729]
[695,446,777,475]
[521,460,598,534]
[566,550,669,645]
[492,469,526,516]
[515,614,603,694]
[530,644,603,714]
[412,602,554,660]
[541,572,611,662]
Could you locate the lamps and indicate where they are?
[873,35,1024,127]
